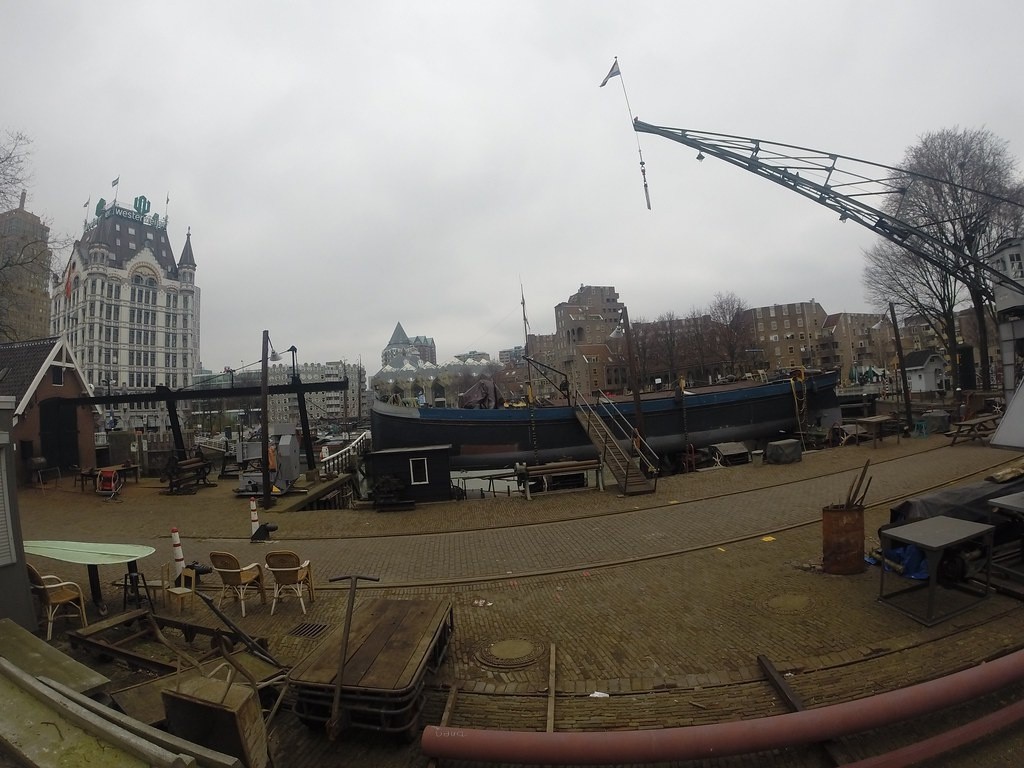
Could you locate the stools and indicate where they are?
[913,419,929,439]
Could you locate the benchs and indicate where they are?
[978,429,997,437]
[944,427,971,437]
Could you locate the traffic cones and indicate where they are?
[171,527,186,579]
[248,496,261,534]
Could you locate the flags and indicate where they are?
[600,55,623,89]
[112,177,119,188]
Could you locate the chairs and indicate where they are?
[26,563,88,640]
[146,560,172,609]
[209,551,267,618]
[167,566,196,616]
[265,550,313,616]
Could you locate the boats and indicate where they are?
[370,365,847,505]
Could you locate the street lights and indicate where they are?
[872,298,914,431]
[260,329,283,508]
[101,378,116,432]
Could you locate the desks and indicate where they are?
[856,415,901,449]
[988,490,1024,581]
[877,516,998,627]
[23,540,156,608]
[93,464,141,488]
[950,415,1001,447]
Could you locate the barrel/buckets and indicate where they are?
[101,470,114,490]
[822,504,865,575]
